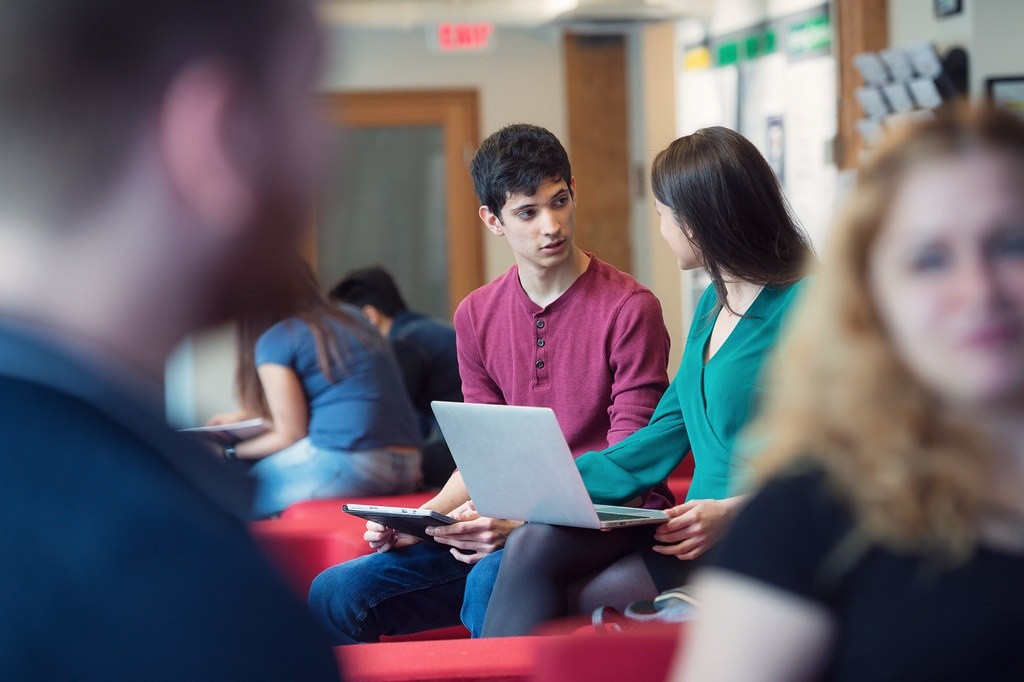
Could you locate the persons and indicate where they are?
[0,0,344,682]
[667,103,1024,682]
[199,123,810,645]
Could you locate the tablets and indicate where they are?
[345,504,458,542]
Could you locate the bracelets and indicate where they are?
[222,442,237,460]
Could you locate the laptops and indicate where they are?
[432,401,675,532]
